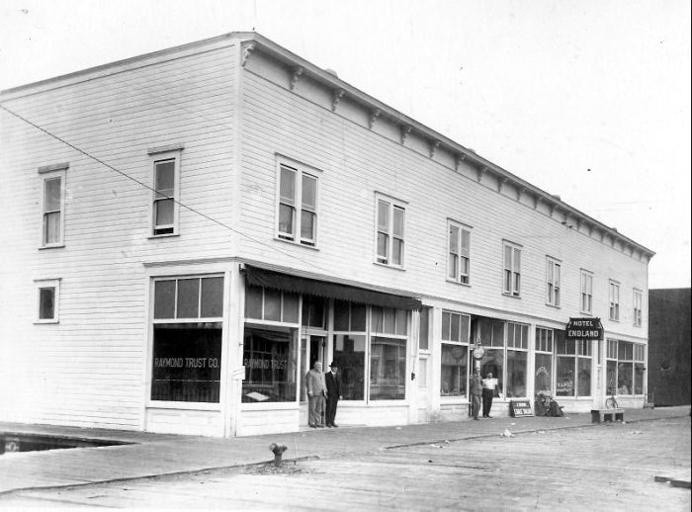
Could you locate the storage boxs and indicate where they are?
[591,408,626,422]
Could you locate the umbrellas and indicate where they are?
[310,424,337,428]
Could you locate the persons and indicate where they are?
[322,361,340,429]
[469,365,483,421]
[480,372,499,418]
[304,361,327,428]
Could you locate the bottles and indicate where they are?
[329,361,340,367]
